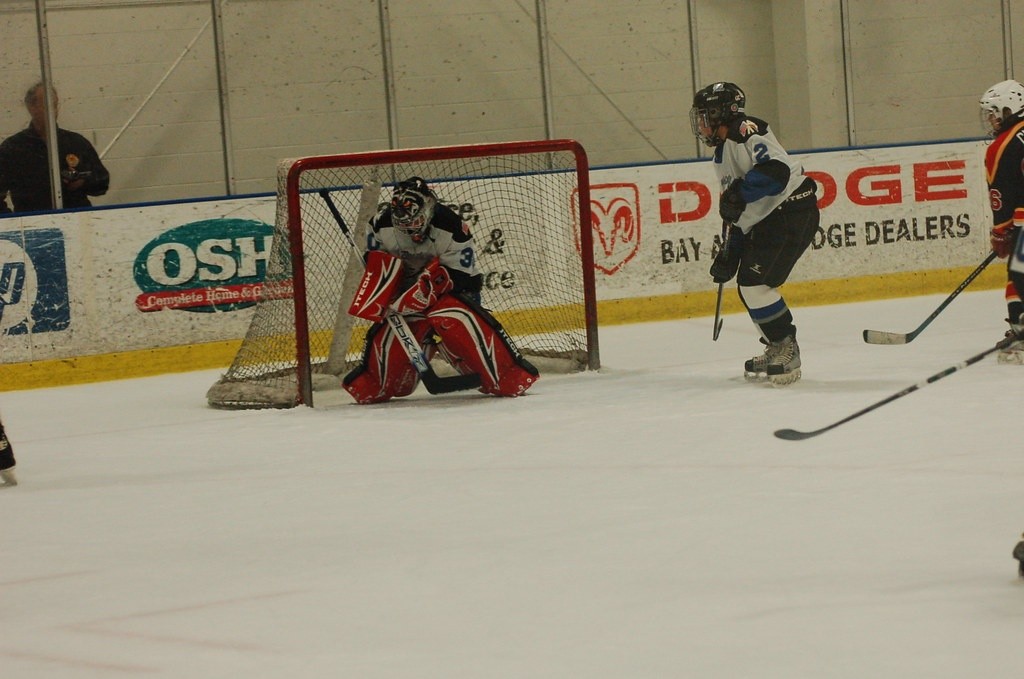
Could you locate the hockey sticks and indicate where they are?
[712,221,732,342]
[318,185,483,396]
[862,251,998,346]
[773,333,1023,441]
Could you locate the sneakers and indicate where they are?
[0,423,17,486]
[998,340,1024,364]
[743,338,775,381]
[765,336,802,387]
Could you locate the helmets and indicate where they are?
[980,79,1024,119]
[391,177,437,224]
[691,82,746,122]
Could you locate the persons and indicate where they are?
[690,83,820,387]
[978,80,1024,362]
[343,176,540,405]
[0,82,110,216]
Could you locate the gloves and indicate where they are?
[388,263,453,316]
[990,231,1012,259]
[709,225,744,283]
[719,183,745,228]
